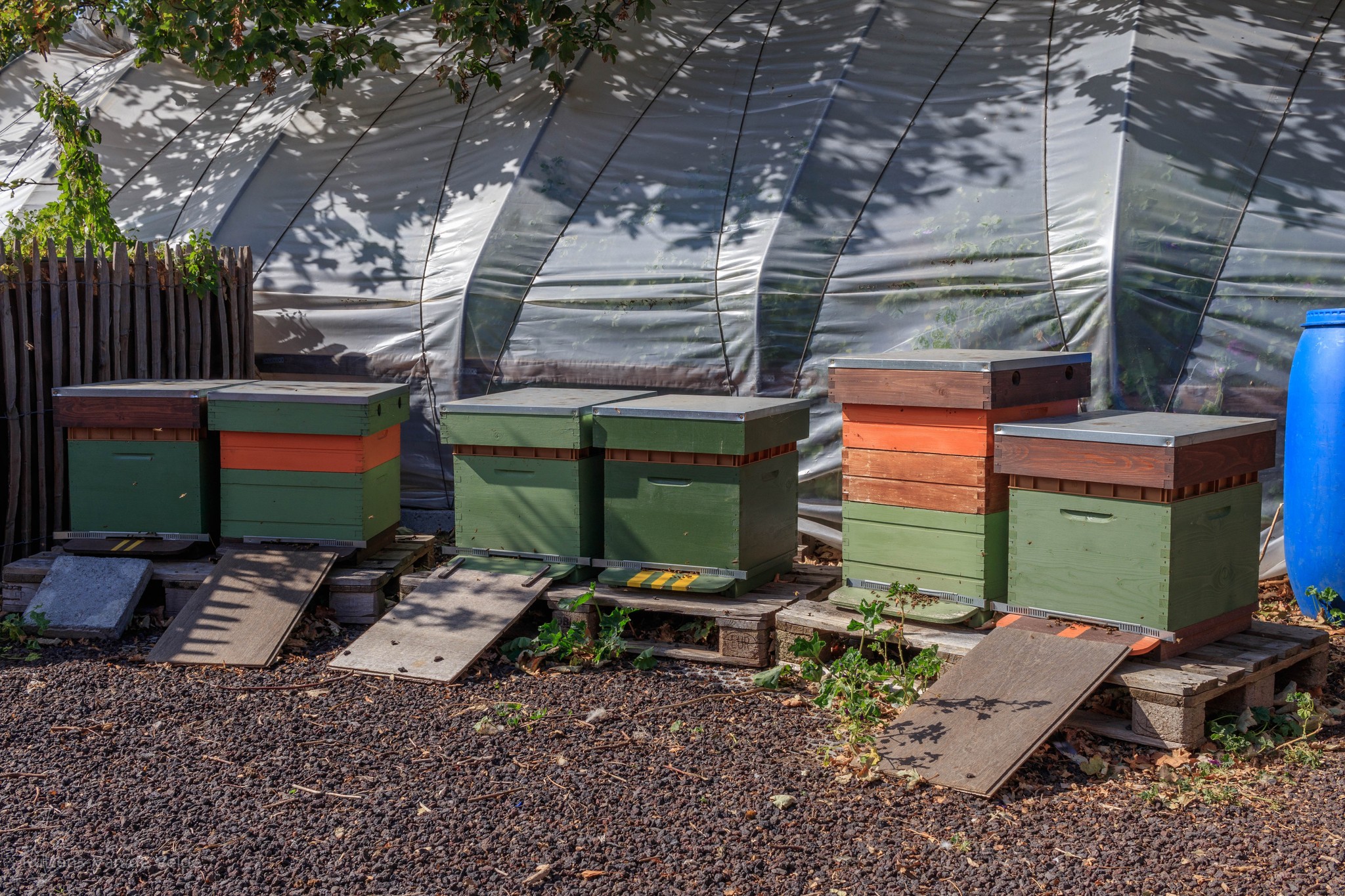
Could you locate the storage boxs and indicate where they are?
[51,376,409,558]
[440,386,809,598]
[826,347,1280,659]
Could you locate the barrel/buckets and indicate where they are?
[1283,308,1345,618]
